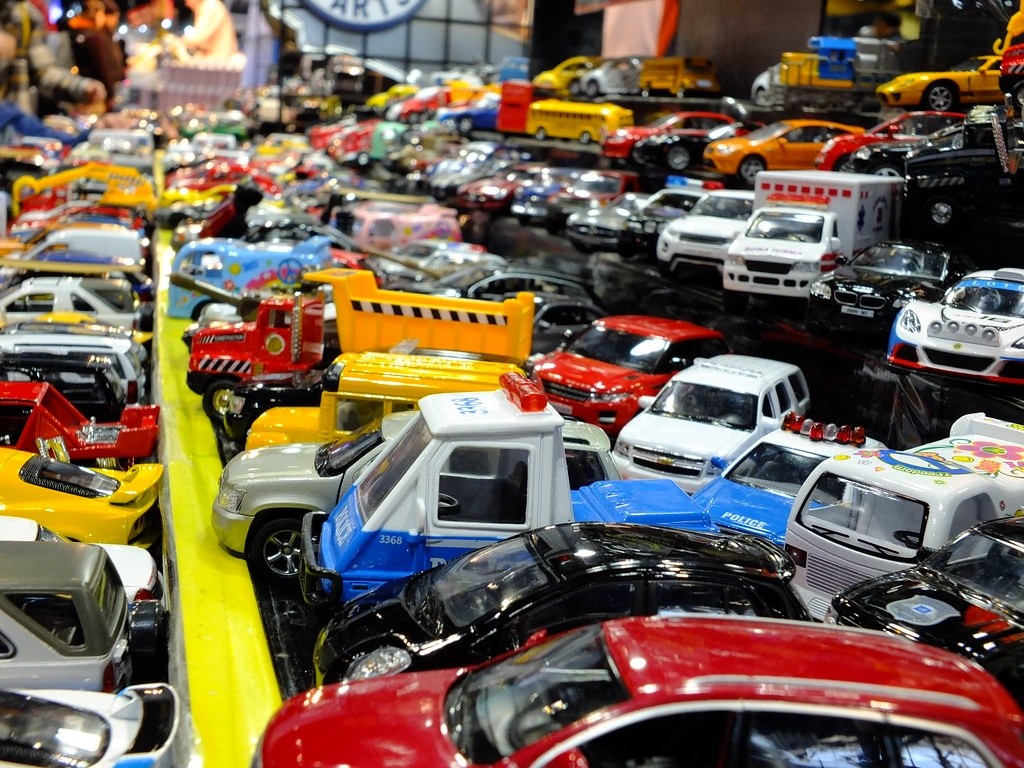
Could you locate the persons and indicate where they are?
[177,0,238,63]
[62,0,128,115]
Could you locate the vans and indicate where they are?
[783,412,1024,625]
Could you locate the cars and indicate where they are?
[819,513,1023,699]
[250,610,1024,768]
[303,519,813,705]
[0,0,1024,768]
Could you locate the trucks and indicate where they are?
[184,265,536,427]
